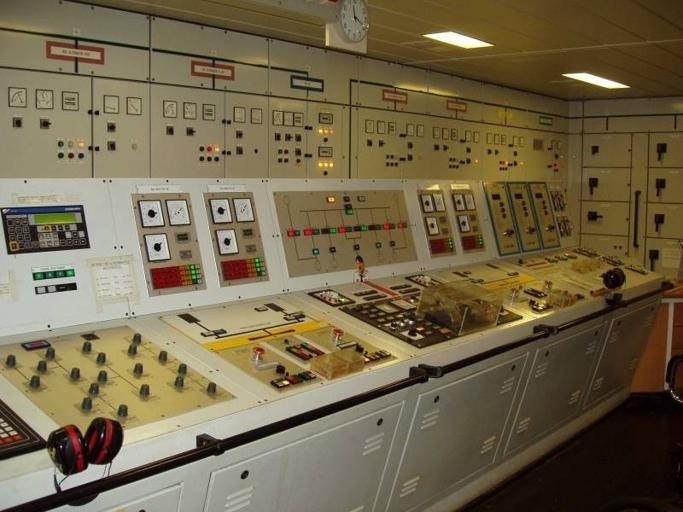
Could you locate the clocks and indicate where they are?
[334,0,370,43]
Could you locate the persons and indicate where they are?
[558,217,573,237]
[353,256,368,283]
[554,191,567,210]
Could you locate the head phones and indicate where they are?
[604,267,626,289]
[46,416,124,475]
